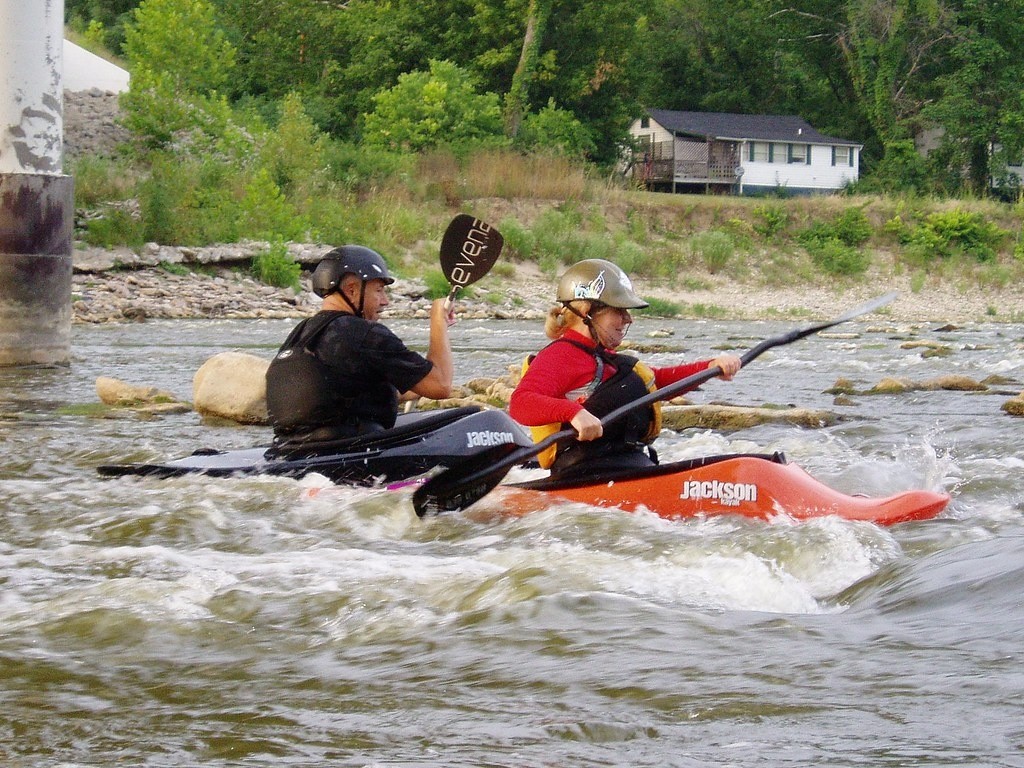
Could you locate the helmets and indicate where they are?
[313,244,395,298]
[555,259,649,309]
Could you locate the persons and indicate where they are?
[265,245,458,438]
[507,258,741,474]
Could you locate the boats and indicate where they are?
[387,454,951,539]
[95,401,538,495]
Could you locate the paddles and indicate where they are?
[408,286,904,524]
[402,210,507,414]
